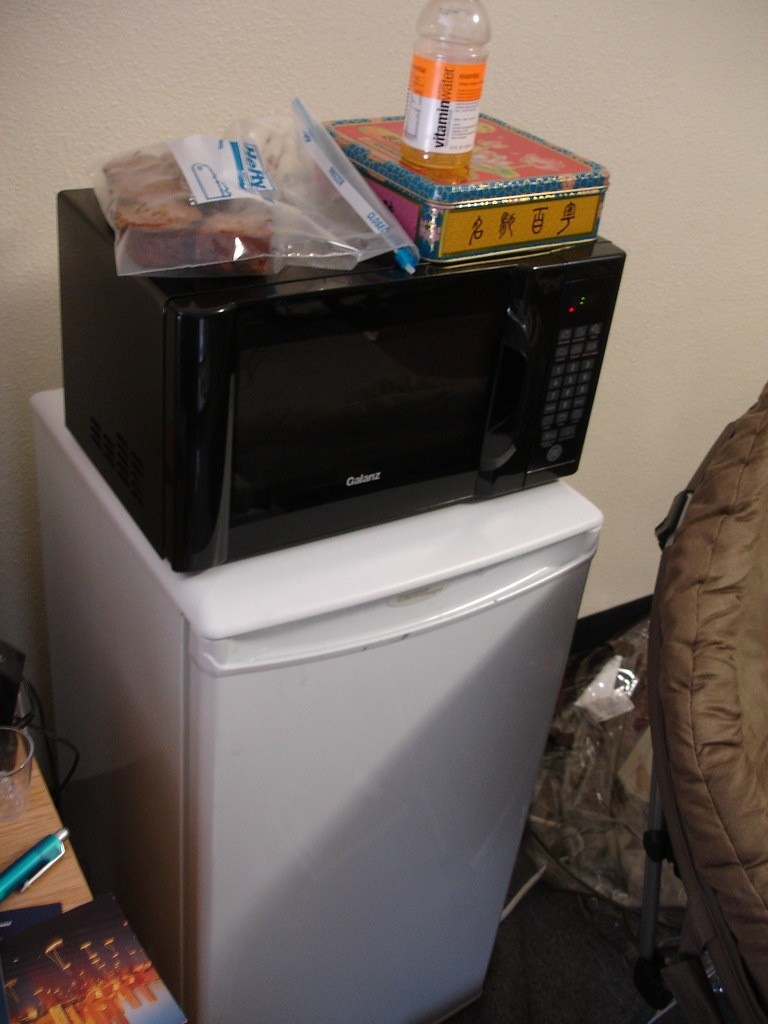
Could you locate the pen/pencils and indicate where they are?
[0,826,72,904]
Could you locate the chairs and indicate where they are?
[629,377,768,1024]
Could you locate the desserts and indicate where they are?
[102,150,273,275]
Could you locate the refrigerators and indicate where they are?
[30,387,607,1024]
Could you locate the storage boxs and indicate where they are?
[321,114,611,263]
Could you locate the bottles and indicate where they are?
[399,0,491,169]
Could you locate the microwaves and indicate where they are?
[57,185,628,574]
